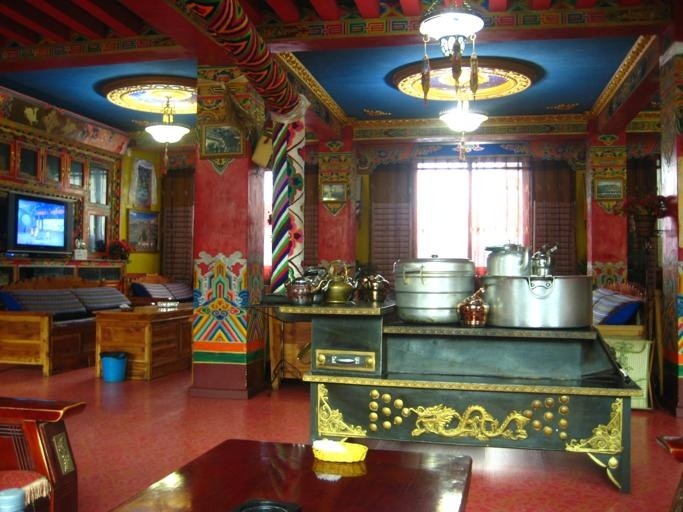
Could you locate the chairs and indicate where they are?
[649,431,683,512]
[0,399,88,510]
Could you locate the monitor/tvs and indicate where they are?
[5,191,74,255]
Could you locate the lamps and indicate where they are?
[417,4,484,101]
[438,91,490,164]
[144,93,187,166]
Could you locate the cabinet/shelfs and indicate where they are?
[279,303,647,495]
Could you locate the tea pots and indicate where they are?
[285,260,389,304]
[528,243,562,276]
[456,286,493,326]
[485,242,536,276]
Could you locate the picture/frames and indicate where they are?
[200,122,245,159]
[321,180,347,203]
[126,208,158,254]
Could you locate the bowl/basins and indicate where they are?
[310,460,368,477]
[312,441,368,463]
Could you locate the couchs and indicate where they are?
[594,312,643,337]
[0,281,166,376]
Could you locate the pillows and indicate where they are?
[1,288,86,317]
[592,294,641,326]
[591,287,619,309]
[73,287,129,311]
[134,282,173,301]
[164,282,193,301]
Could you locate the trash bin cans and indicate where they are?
[100,352,128,383]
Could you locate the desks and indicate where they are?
[597,336,652,412]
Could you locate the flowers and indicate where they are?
[104,238,132,259]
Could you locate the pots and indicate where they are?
[476,272,597,329]
[394,253,476,326]
